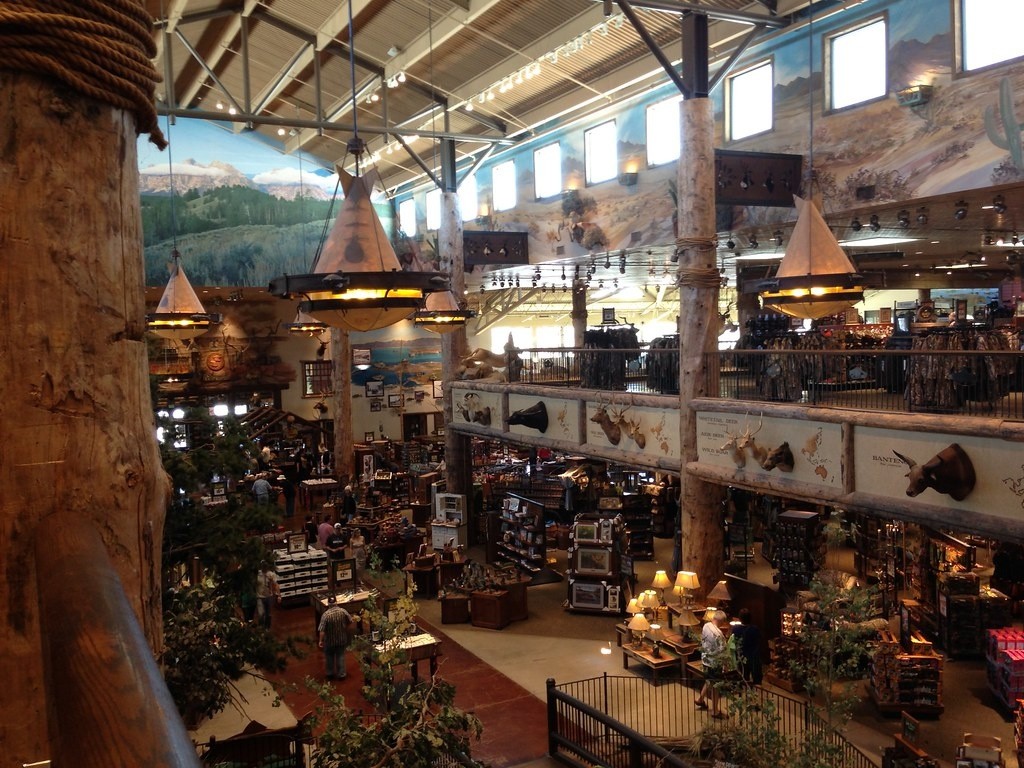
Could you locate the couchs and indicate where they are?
[813,618,889,682]
[795,567,860,632]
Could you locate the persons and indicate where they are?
[318,516,334,558]
[318,597,351,680]
[326,523,347,574]
[724,487,747,529]
[255,561,281,634]
[252,475,272,511]
[295,445,305,482]
[319,443,332,475]
[341,486,357,525]
[436,457,447,475]
[277,475,296,520]
[695,610,729,719]
[350,529,366,580]
[303,513,319,551]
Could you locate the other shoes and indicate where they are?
[326,674,332,679]
[746,704,752,711]
[340,675,345,679]
[265,627,270,631]
[752,704,760,712]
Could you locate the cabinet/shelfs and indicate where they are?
[778,510,819,591]
[599,495,655,561]
[418,472,438,504]
[865,629,945,717]
[430,494,468,551]
[354,449,376,481]
[491,492,564,587]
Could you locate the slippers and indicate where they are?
[711,710,726,720]
[694,700,708,710]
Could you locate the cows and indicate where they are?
[456,392,491,426]
[892,443,976,501]
[504,401,548,433]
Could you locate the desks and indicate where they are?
[661,634,704,687]
[402,555,528,630]
[668,601,707,636]
[346,515,392,543]
[309,583,379,645]
[302,478,338,511]
[365,539,407,570]
[686,661,743,702]
[411,501,431,527]
[397,533,427,558]
[621,642,681,689]
[356,505,381,520]
[357,625,443,713]
[616,623,629,647]
[623,614,682,647]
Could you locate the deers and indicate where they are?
[590,386,646,449]
[720,411,768,469]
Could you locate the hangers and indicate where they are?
[582,320,1021,348]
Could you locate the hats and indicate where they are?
[345,485,352,492]
[334,524,341,528]
[328,594,336,603]
[277,474,286,481]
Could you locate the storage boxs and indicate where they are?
[938,570,1024,709]
[272,544,329,599]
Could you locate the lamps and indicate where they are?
[143,0,223,340]
[228,104,236,115]
[479,249,628,295]
[895,84,930,106]
[267,0,475,331]
[624,570,733,659]
[479,15,625,104]
[742,0,868,319]
[475,215,491,228]
[559,189,579,205]
[870,194,1024,265]
[617,173,638,187]
[216,99,224,109]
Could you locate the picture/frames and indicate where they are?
[371,402,381,412]
[351,347,372,365]
[608,586,619,610]
[388,394,404,408]
[365,380,385,398]
[574,521,598,545]
[415,391,424,400]
[599,518,614,545]
[433,380,443,398]
[365,432,374,443]
[572,546,613,577]
[569,579,607,612]
